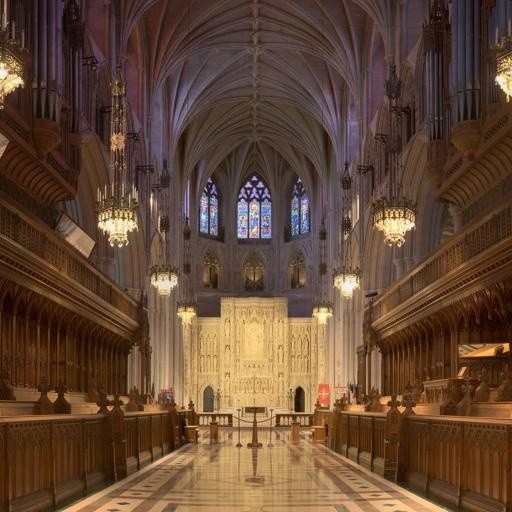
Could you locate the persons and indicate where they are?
[178,405,188,443]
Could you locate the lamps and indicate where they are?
[312,216,335,327]
[95,78,139,250]
[330,159,362,299]
[370,65,418,250]
[493,12,512,102]
[0,17,29,111]
[150,157,198,327]
